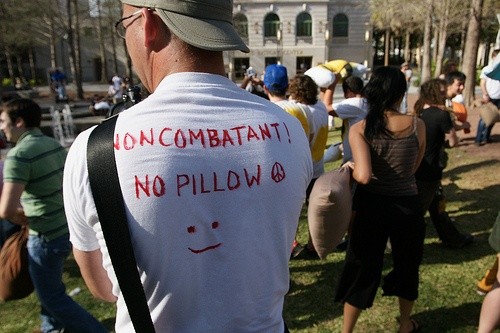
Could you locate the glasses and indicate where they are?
[114,8,152,40]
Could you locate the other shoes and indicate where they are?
[290,243,320,260]
[449,234,477,250]
[476,252,498,295]
[401,320,421,333]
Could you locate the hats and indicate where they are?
[247,66,257,77]
[264,63,289,90]
[120,0,251,53]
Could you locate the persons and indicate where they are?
[320,74,370,165]
[50,69,67,100]
[0,92,111,333]
[335,67,425,332]
[240,65,328,259]
[438,60,471,131]
[477,212,500,333]
[401,62,413,115]
[62,0,314,333]
[92,74,131,114]
[475,50,500,146]
[386,78,473,250]
